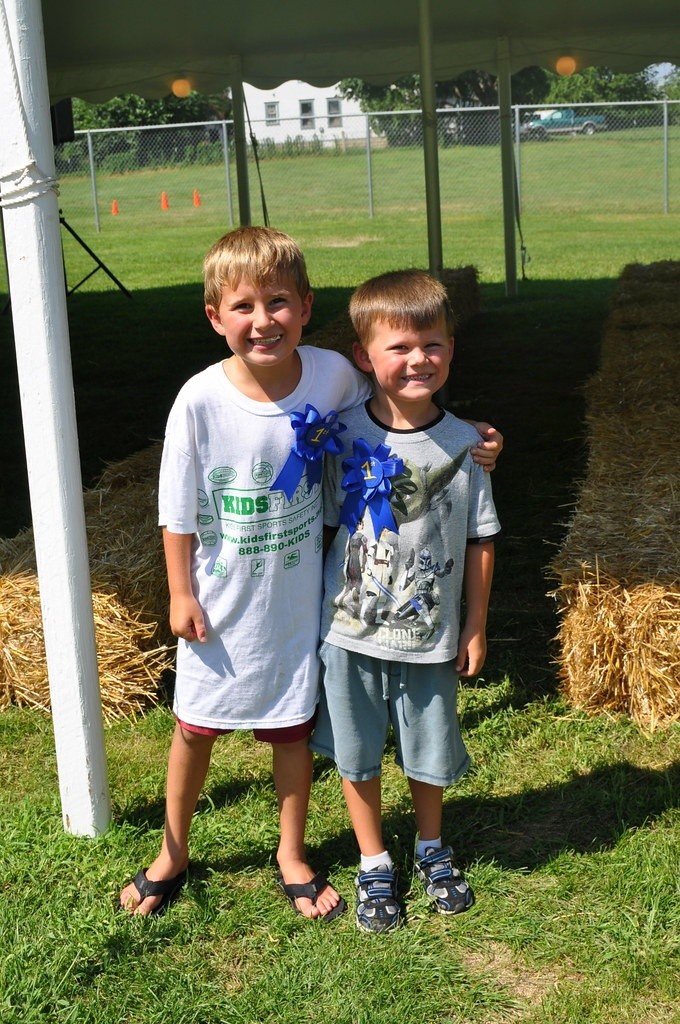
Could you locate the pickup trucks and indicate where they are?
[527,107,608,135]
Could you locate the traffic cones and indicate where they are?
[193,189,202,206]
[161,192,169,210]
[111,200,119,215]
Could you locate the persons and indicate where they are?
[115,225,505,934]
[320,267,499,930]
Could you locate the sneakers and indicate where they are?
[355,864,404,934]
[415,846,475,915]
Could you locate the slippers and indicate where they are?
[270,851,346,924]
[118,860,192,916]
